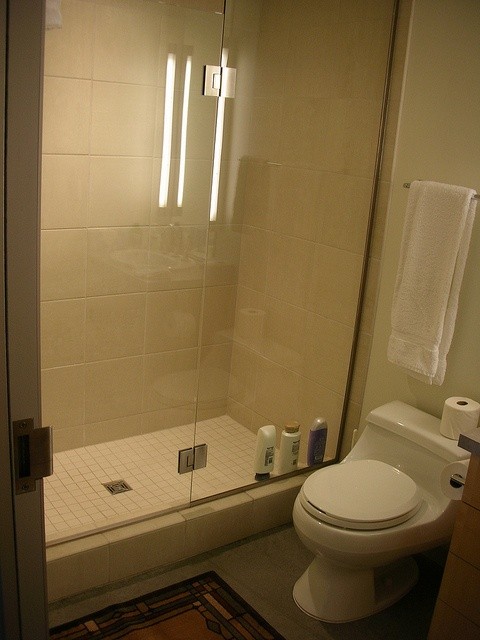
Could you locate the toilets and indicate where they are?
[292,400,471,627]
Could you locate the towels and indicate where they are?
[387,179,477,384]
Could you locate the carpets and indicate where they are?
[48,570,286,639]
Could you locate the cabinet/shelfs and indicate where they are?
[426,453,480,639]
